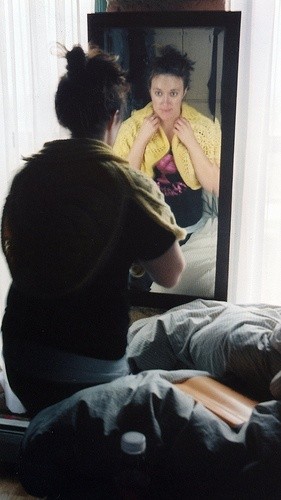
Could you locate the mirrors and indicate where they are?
[87,10,242,308]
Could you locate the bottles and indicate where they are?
[100,431,173,500]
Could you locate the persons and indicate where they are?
[1,45,185,427]
[112,45,222,294]
